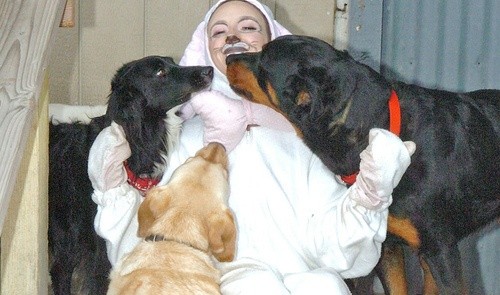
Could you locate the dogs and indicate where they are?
[46,55,214,295]
[104,142,236,295]
[225,34,500,295]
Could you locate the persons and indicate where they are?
[88,0,417,295]
